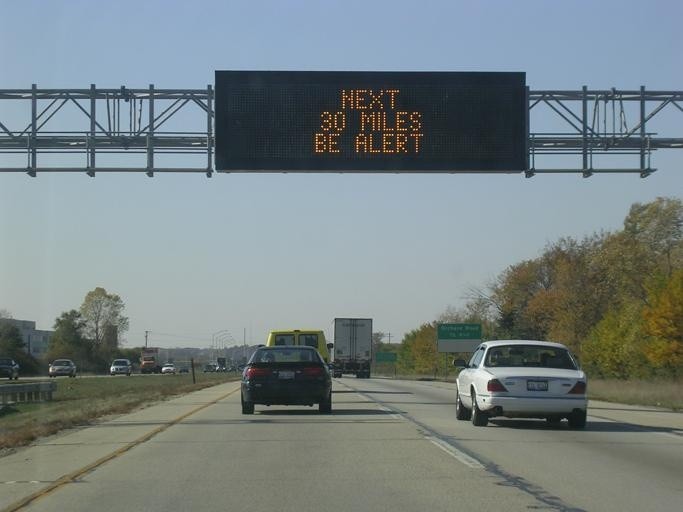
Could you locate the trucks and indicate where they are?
[140,356,160,374]
[331,318,372,378]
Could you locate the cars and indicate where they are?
[241,346,332,414]
[0,357,19,380]
[162,363,189,374]
[203,365,236,373]
[49,359,76,377]
[452,340,587,426]
[109,359,132,376]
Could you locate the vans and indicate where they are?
[266,329,333,364]
